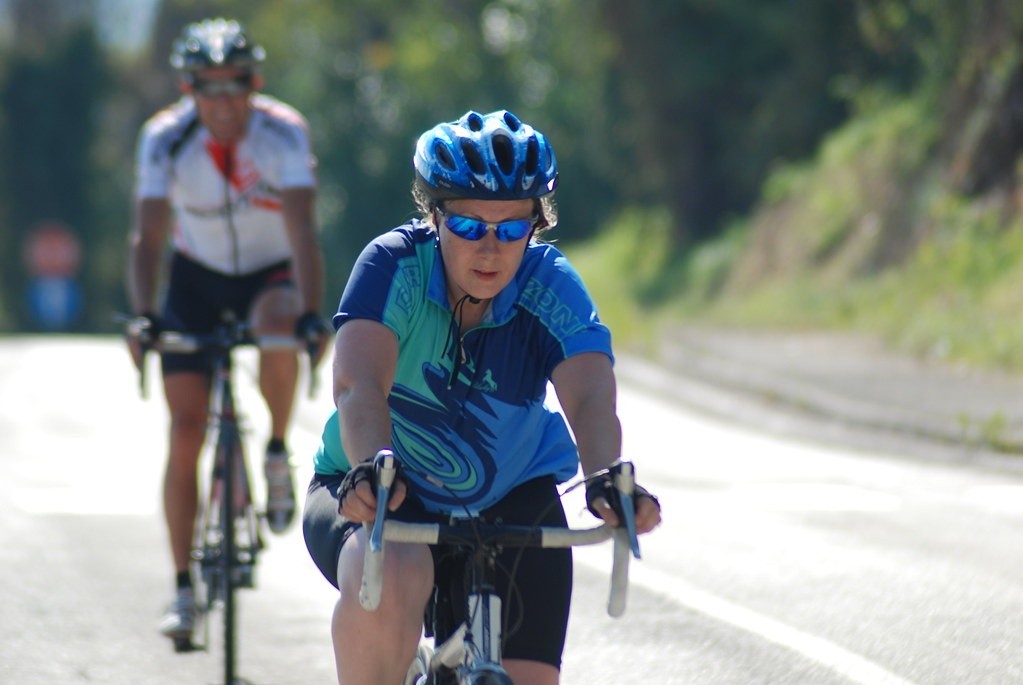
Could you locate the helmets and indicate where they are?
[169,16,267,71]
[414,109,559,201]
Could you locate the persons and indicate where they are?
[23,220,83,332]
[126,19,332,638]
[302,108,660,685]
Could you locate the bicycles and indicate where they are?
[359,450,641,685]
[134,333,318,685]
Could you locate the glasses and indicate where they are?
[435,206,539,242]
[190,74,252,95]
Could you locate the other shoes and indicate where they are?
[158,588,195,638]
[264,461,295,533]
